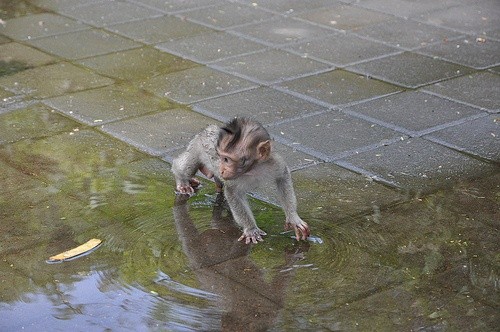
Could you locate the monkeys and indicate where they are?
[172,116,311,245]
